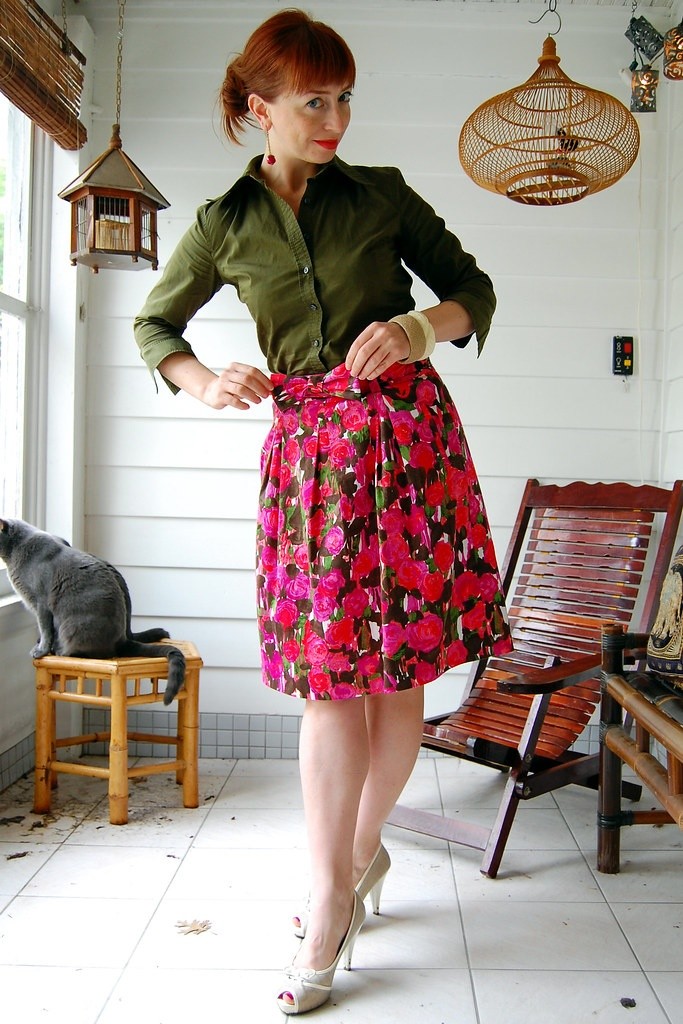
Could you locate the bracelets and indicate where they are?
[388,310,436,364]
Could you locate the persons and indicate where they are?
[133,7,515,1015]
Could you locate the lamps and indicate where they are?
[624,0,683,113]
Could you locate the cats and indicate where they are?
[36,536,171,643]
[0,518,186,707]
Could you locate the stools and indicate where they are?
[33,637,203,825]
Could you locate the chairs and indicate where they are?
[380,478,683,878]
[597,621,683,874]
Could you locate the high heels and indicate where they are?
[291,843,390,939]
[275,887,367,1014]
